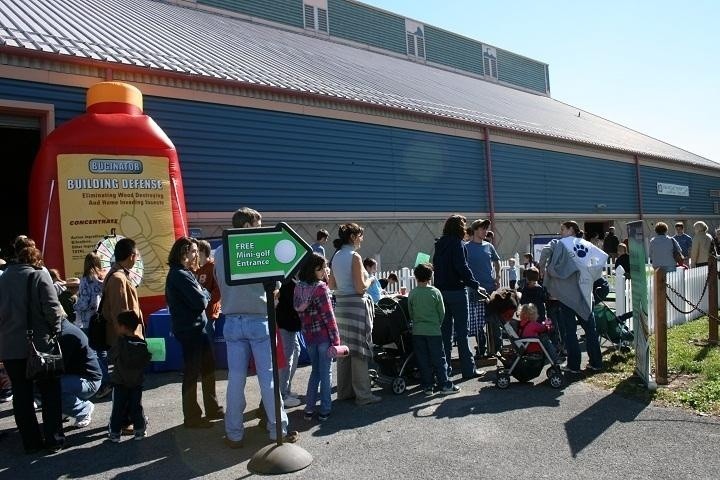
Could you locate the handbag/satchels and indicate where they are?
[671,238,685,267]
[87,310,109,351]
[326,343,350,358]
[25,343,66,381]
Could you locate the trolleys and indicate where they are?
[590,289,635,370]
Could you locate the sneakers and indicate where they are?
[283,389,301,407]
[24,444,65,454]
[183,408,225,429]
[73,398,95,428]
[95,382,113,398]
[271,429,300,444]
[111,428,148,444]
[424,381,463,396]
[560,364,581,374]
[0,389,12,403]
[221,432,244,450]
[302,410,332,422]
[585,362,603,371]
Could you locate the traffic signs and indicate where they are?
[220,220,312,286]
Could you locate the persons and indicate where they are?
[648,220,689,273]
[690,220,716,269]
[672,221,693,268]
[714,228,720,256]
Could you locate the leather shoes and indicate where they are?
[465,368,487,377]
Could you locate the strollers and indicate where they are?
[371,295,425,395]
[472,282,565,389]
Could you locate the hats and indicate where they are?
[61,278,80,288]
[470,218,491,230]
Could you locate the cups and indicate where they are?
[544,318,554,332]
[327,342,350,357]
[399,286,407,297]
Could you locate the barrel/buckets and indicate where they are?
[414,251,431,268]
[149,305,228,370]
